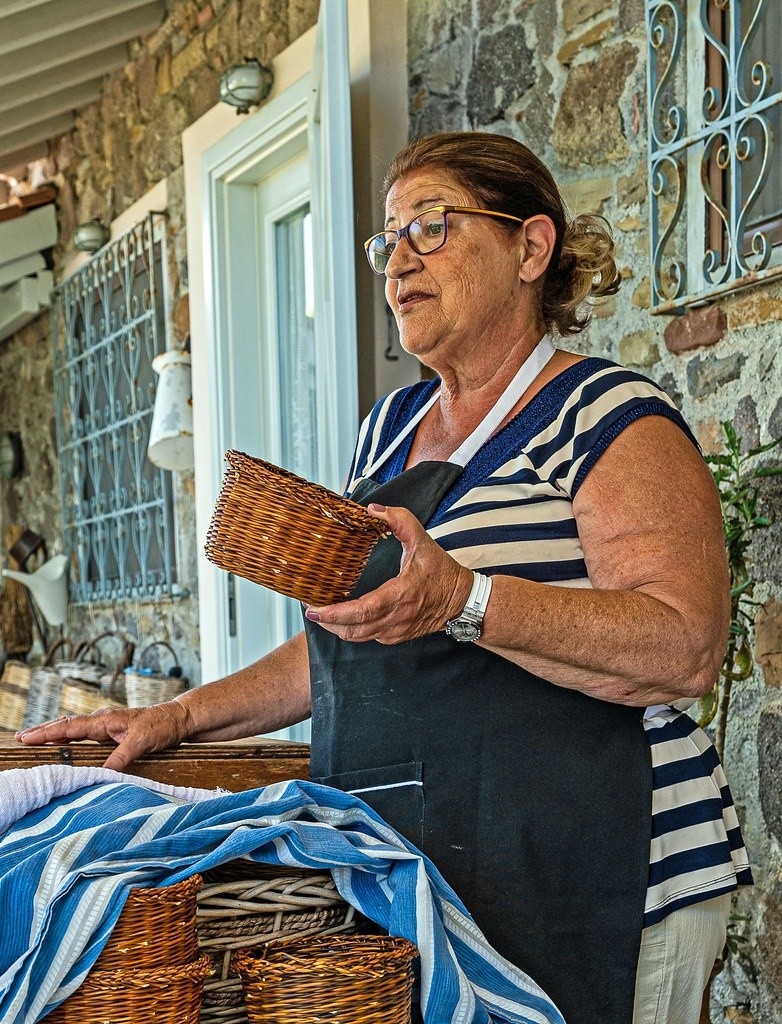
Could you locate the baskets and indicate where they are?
[204,448,390,607]
[0,627,188,733]
[36,874,417,1024]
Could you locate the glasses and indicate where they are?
[362,206,525,276]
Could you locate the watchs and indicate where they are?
[446,570,493,643]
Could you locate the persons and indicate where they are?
[12,131,732,1024]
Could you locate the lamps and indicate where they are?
[72,222,110,252]
[216,54,278,114]
